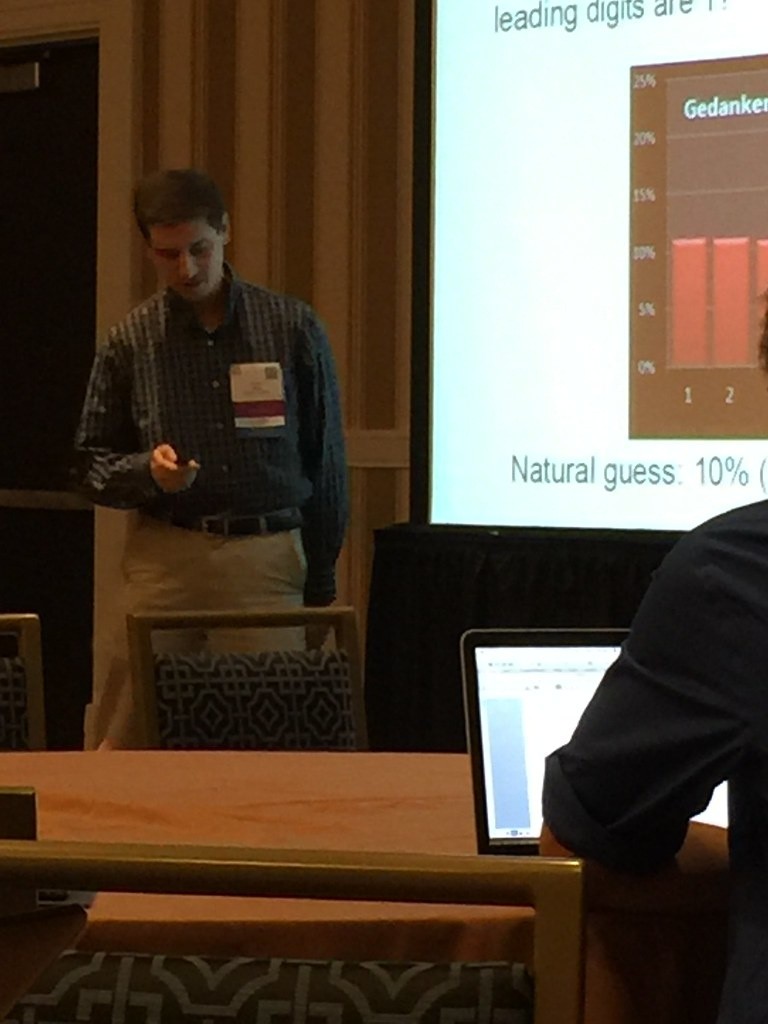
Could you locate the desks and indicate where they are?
[0,753,587,1024]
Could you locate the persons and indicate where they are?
[67,166,349,750]
[538,500,767,1024]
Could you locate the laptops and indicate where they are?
[460,628,729,856]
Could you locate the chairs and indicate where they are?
[0,613,46,750]
[121,607,356,751]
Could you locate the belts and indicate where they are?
[137,504,303,538]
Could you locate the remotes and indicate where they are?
[176,454,194,466]
